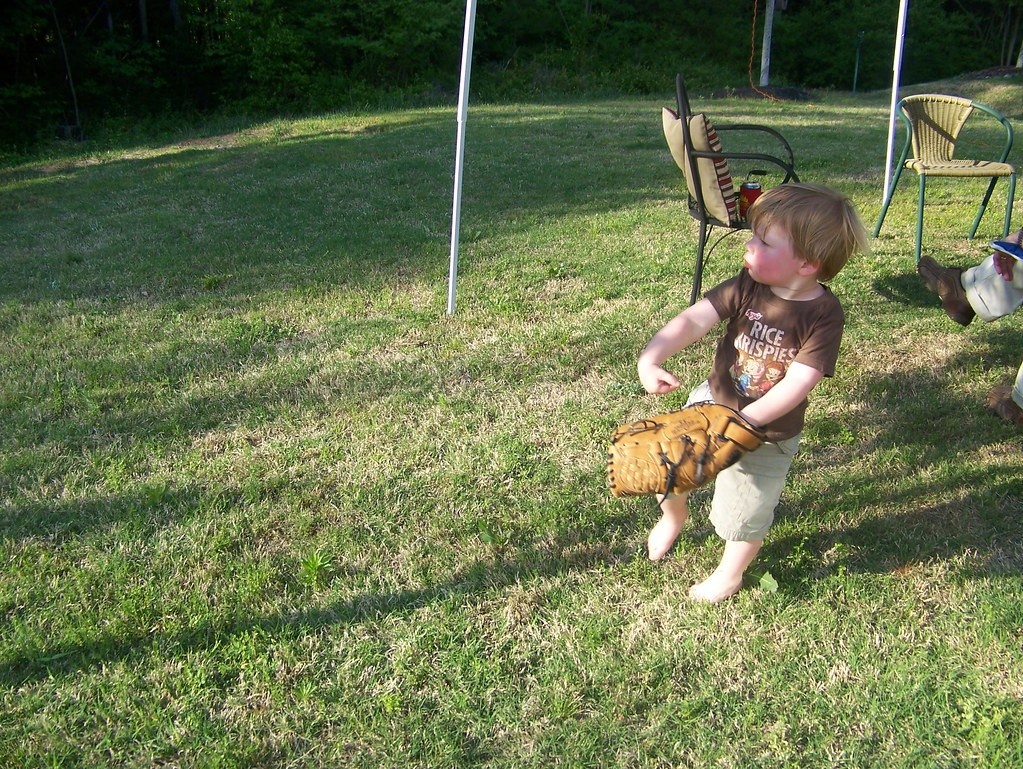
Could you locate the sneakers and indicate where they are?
[917,255,973,326]
[987,385,1023,434]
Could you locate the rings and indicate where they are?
[1000,255,1007,260]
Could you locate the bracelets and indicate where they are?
[1017,227,1023,250]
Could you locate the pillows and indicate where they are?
[659,105,735,227]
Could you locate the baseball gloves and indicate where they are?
[606,399,769,520]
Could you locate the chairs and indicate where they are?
[874,93,1017,272]
[675,72,801,307]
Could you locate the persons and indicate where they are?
[636,181,873,606]
[917,226,1023,438]
